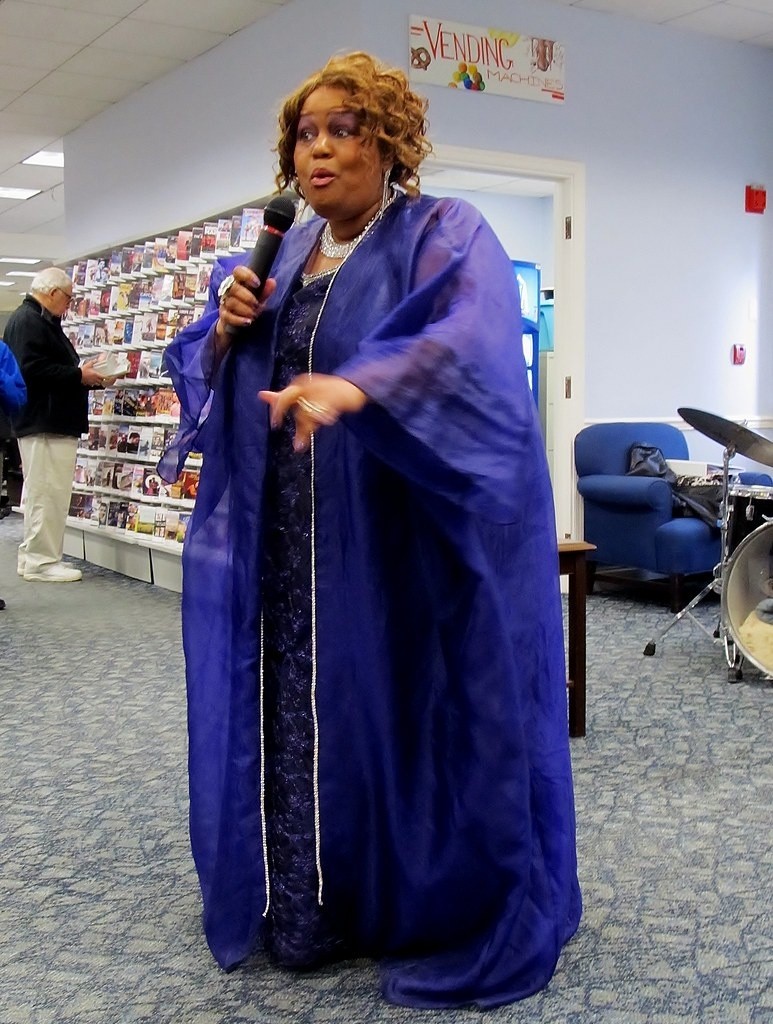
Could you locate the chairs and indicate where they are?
[574,421,773,613]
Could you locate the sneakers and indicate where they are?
[18,561,83,582]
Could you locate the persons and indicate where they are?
[100,266,111,284]
[127,504,137,531]
[90,258,108,283]
[147,479,156,495]
[153,435,163,449]
[0,335,27,610]
[118,501,127,528]
[126,432,140,453]
[3,267,118,582]
[154,49,582,1012]
[99,503,107,524]
[151,478,159,491]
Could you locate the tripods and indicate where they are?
[644,443,744,683]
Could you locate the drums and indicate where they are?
[727,484,773,559]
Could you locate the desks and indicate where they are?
[557,538,597,739]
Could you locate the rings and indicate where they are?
[218,275,235,297]
[220,294,228,305]
[296,396,328,413]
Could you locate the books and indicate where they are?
[55,208,270,542]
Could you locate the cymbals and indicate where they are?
[677,407,773,467]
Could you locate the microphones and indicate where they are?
[232,196,296,329]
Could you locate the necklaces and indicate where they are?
[318,192,395,258]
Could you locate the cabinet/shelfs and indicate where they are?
[11,180,315,594]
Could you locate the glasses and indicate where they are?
[55,285,74,305]
